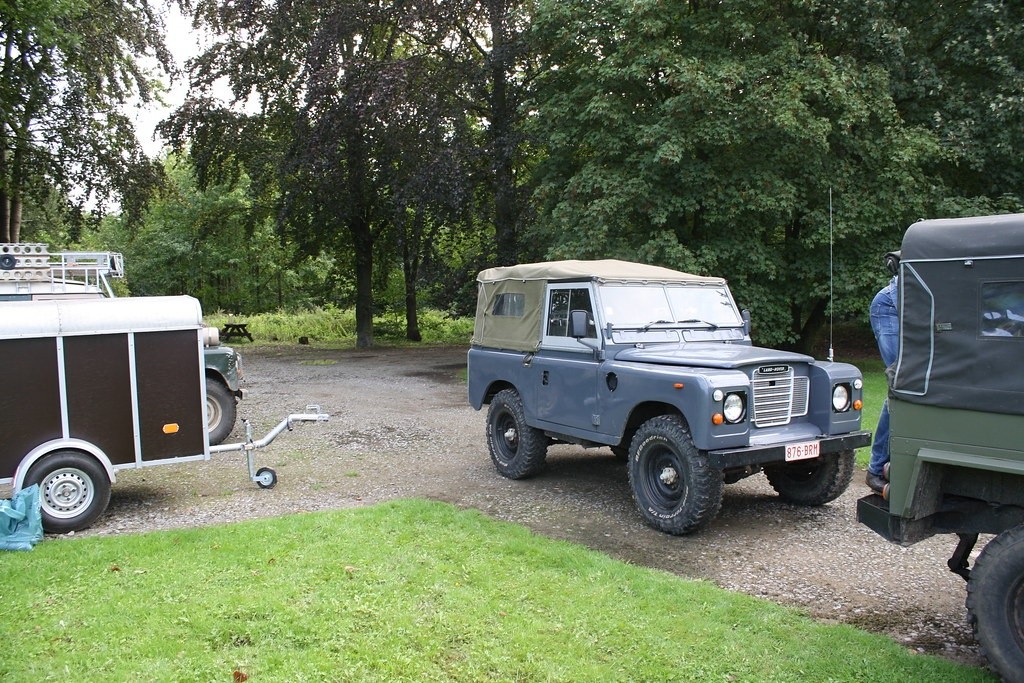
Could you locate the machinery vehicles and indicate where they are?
[1,242,212,535]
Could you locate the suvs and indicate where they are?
[201,326,244,446]
[858,211,1023,681]
[466,261,875,536]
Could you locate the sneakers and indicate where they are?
[865,470,890,496]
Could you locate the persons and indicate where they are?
[865,278,900,494]
